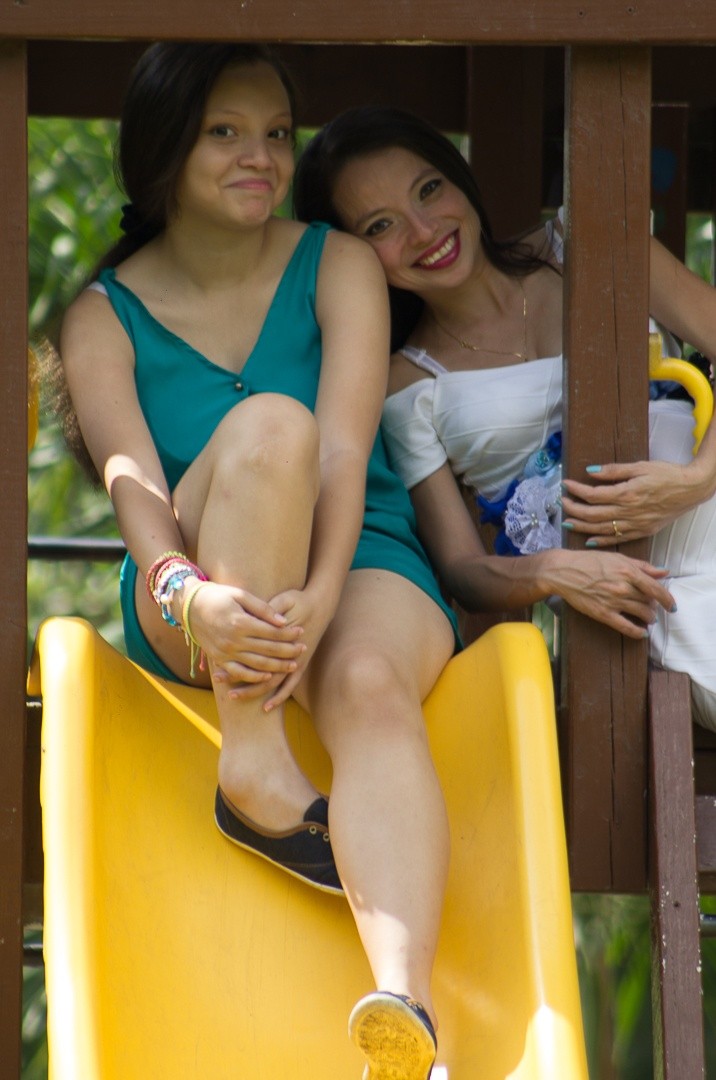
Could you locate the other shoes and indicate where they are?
[215,783,347,899]
[348,991,437,1080]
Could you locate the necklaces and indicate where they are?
[431,276,529,361]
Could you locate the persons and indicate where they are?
[59,38,463,1080]
[292,106,716,733]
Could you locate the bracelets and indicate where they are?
[145,551,209,678]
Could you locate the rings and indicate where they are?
[613,521,623,537]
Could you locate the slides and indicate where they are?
[28,615,591,1080]
